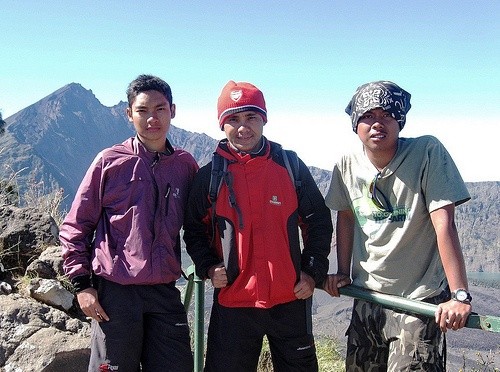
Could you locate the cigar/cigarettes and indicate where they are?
[446,319,450,324]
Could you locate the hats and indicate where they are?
[217,81,267,131]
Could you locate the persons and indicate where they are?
[325,81,472,372]
[58,74,199,372]
[182,80,333,372]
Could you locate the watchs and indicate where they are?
[451,288,472,303]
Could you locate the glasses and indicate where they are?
[369,172,392,214]
